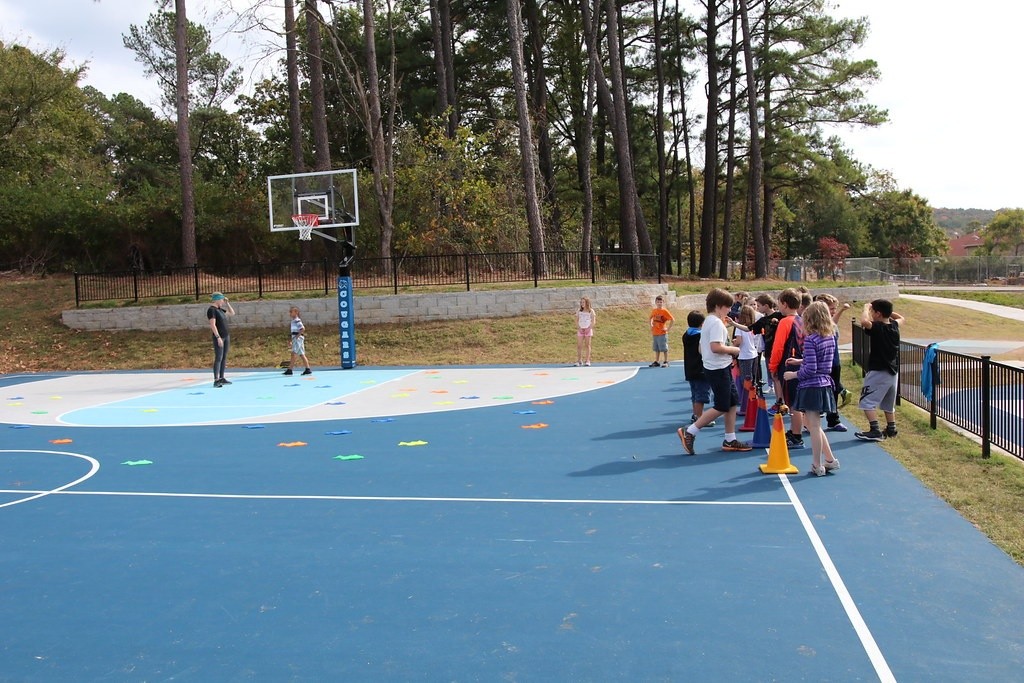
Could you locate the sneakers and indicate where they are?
[218,378,232,385]
[854,429,883,441]
[822,459,840,471]
[214,380,222,388]
[282,369,293,375]
[690,414,716,427]
[649,361,660,367]
[762,385,775,396]
[301,368,312,376]
[841,389,852,405]
[785,429,806,449]
[811,463,826,477]
[881,427,898,439]
[678,425,696,455]
[661,362,668,367]
[721,439,752,451]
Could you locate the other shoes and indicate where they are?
[736,410,746,416]
[819,412,825,418]
[584,362,590,366]
[767,405,785,416]
[574,362,583,367]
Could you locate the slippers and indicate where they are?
[827,423,847,432]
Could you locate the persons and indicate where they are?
[574,298,596,366]
[676,287,753,454]
[283,306,312,375]
[649,295,675,368]
[206,292,236,388]
[681,286,852,449]
[853,299,905,442]
[782,301,840,478]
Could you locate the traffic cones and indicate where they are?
[760,414,799,474]
[732,365,758,431]
[746,397,771,448]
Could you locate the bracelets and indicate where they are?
[216,335,220,338]
